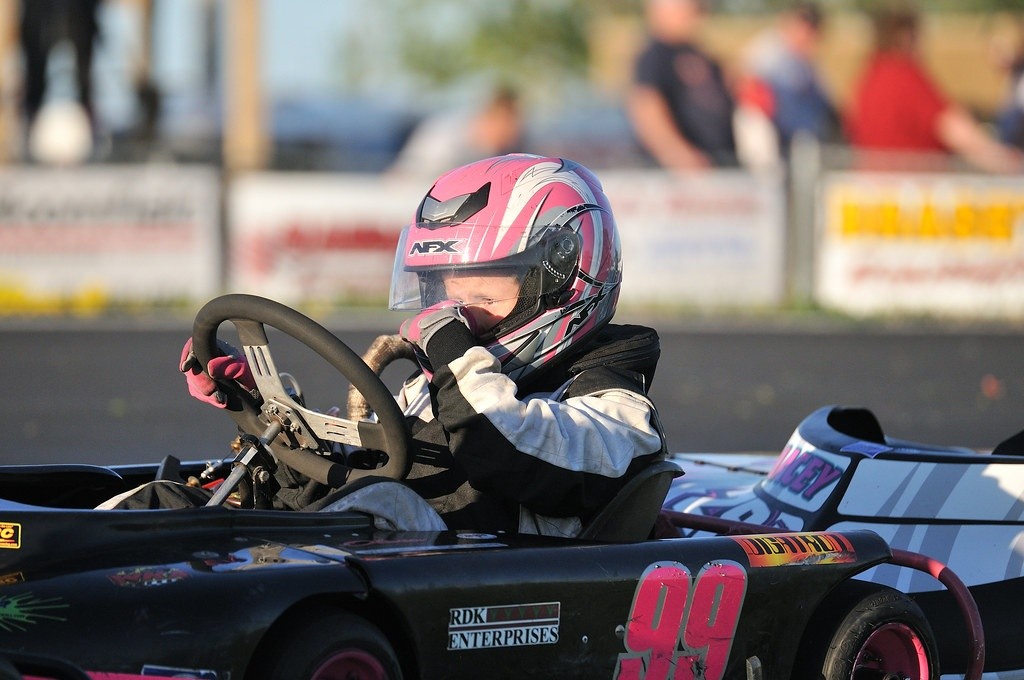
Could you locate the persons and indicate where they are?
[386,87,526,175]
[620,0,1024,173]
[94,153,668,537]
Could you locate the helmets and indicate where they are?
[389,152,623,383]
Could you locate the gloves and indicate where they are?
[399,300,477,384]
[179,336,262,409]
